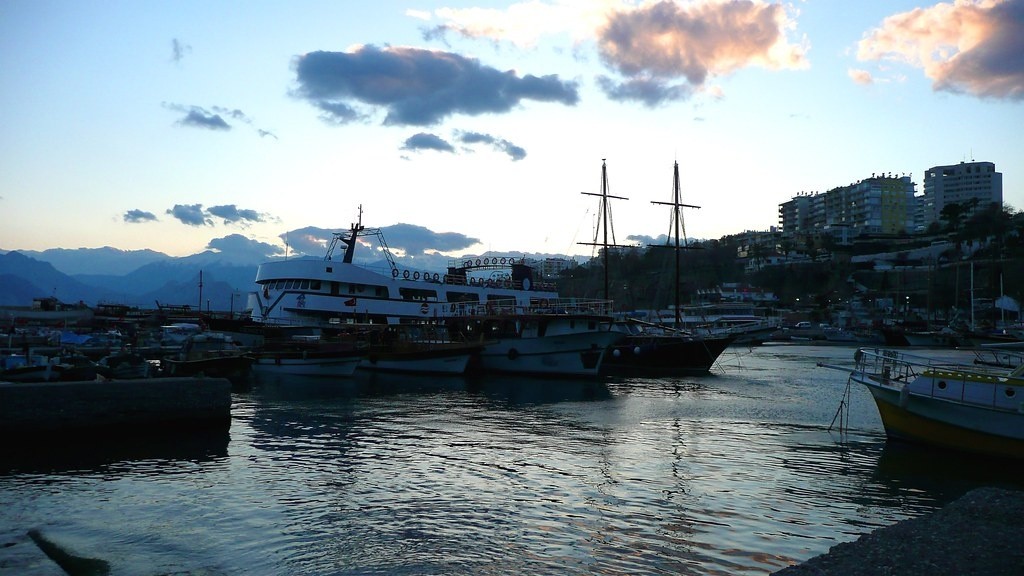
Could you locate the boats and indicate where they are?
[815,346,1024,460]
[0,155,1024,438]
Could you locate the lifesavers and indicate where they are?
[413,271,420,279]
[548,285,554,290]
[471,277,475,283]
[496,279,502,286]
[486,302,492,312]
[433,273,439,281]
[403,269,410,278]
[479,278,484,285]
[500,258,506,264]
[424,272,430,280]
[487,278,492,285]
[508,349,517,360]
[463,260,473,267]
[504,280,511,287]
[450,303,456,312]
[540,298,549,307]
[391,269,399,278]
[484,258,489,264]
[509,258,515,265]
[491,257,498,264]
[475,259,480,266]
[540,283,546,290]
